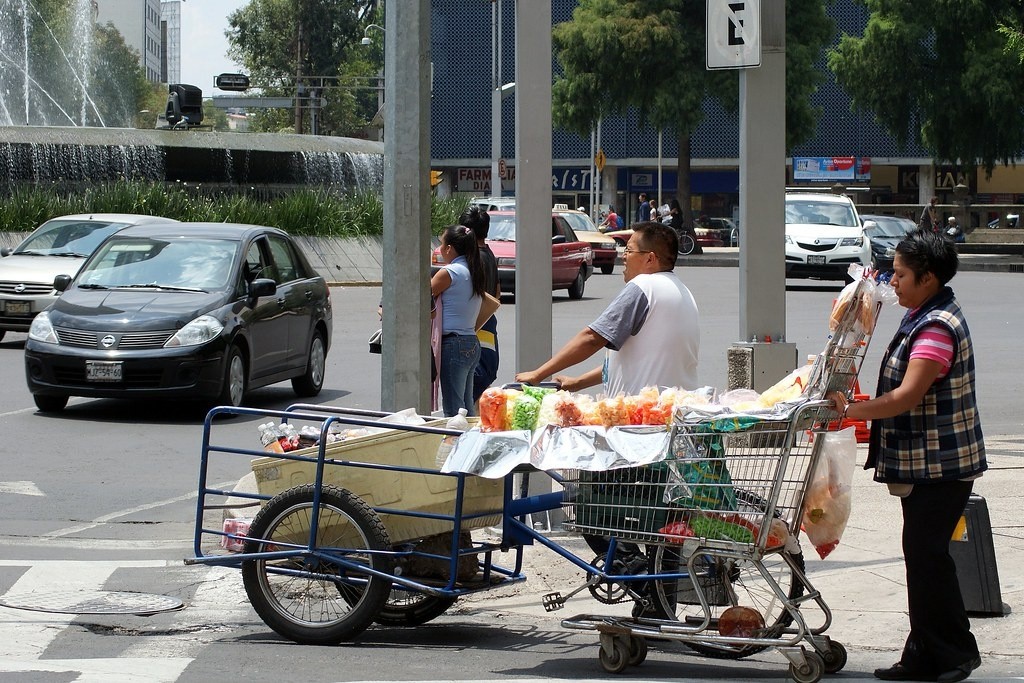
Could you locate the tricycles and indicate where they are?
[184,383,806,659]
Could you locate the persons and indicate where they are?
[593,204,623,231]
[514,222,700,644]
[824,227,989,683]
[378,206,502,418]
[638,193,714,228]
[919,196,961,241]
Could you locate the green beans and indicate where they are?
[512,395,541,432]
[689,517,753,542]
[522,385,549,404]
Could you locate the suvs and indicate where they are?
[470,196,514,210]
[695,219,739,246]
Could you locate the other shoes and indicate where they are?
[938,650,982,683]
[874,661,938,683]
[631,581,650,617]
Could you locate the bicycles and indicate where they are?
[675,228,696,255]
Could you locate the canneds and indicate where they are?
[222,519,254,550]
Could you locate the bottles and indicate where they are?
[288,423,305,447]
[257,424,285,454]
[807,354,818,365]
[302,425,372,447]
[436,407,468,470]
[266,422,292,453]
[278,422,307,451]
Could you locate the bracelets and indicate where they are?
[843,401,850,419]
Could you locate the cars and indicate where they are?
[428,206,597,299]
[604,218,724,245]
[25,220,331,413]
[786,191,878,286]
[554,204,618,275]
[0,212,179,337]
[857,214,921,269]
[988,214,1019,228]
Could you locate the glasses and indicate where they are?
[623,247,661,261]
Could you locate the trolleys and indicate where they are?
[561,392,847,683]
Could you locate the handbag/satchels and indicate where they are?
[799,443,850,559]
[475,289,501,332]
[616,216,624,229]
